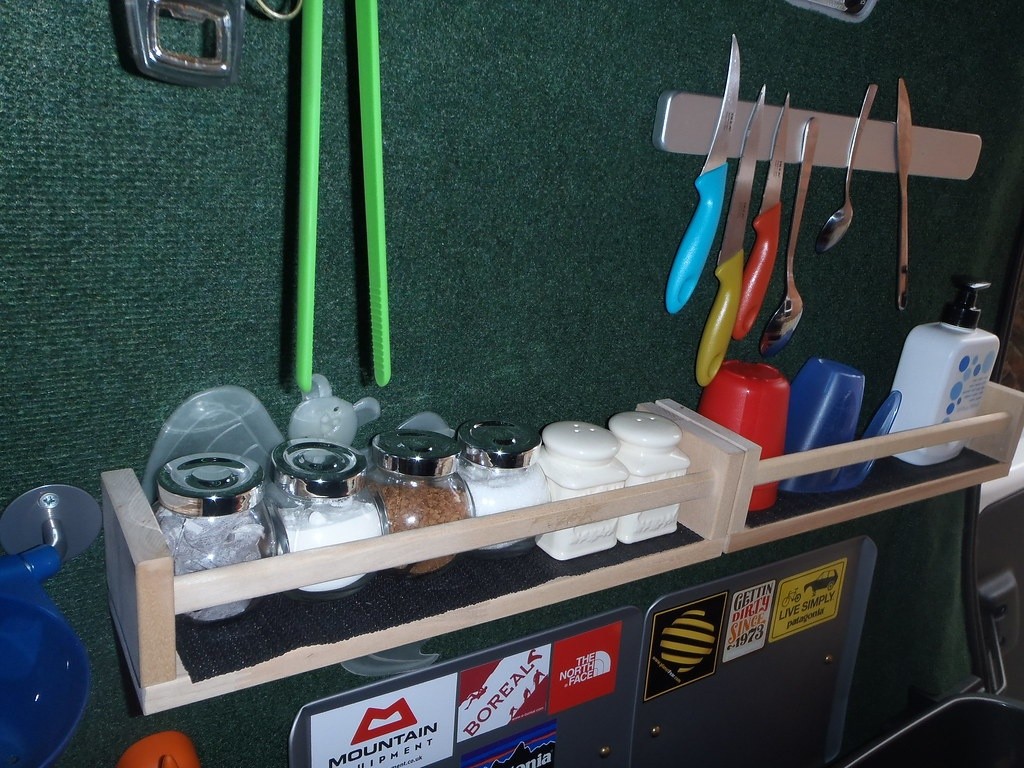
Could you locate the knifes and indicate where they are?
[666,33,740,314]
[895,77,912,311]
[695,84,766,387]
[731,90,789,340]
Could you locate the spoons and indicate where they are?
[815,84,878,253]
[759,118,803,357]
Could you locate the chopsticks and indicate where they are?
[293,0,391,391]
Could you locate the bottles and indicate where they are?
[529,422,628,561]
[369,429,475,580]
[453,418,552,558]
[151,452,275,623]
[608,412,690,544]
[263,438,391,600]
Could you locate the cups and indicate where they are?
[699,359,790,512]
[778,357,902,493]
[116,731,200,768]
